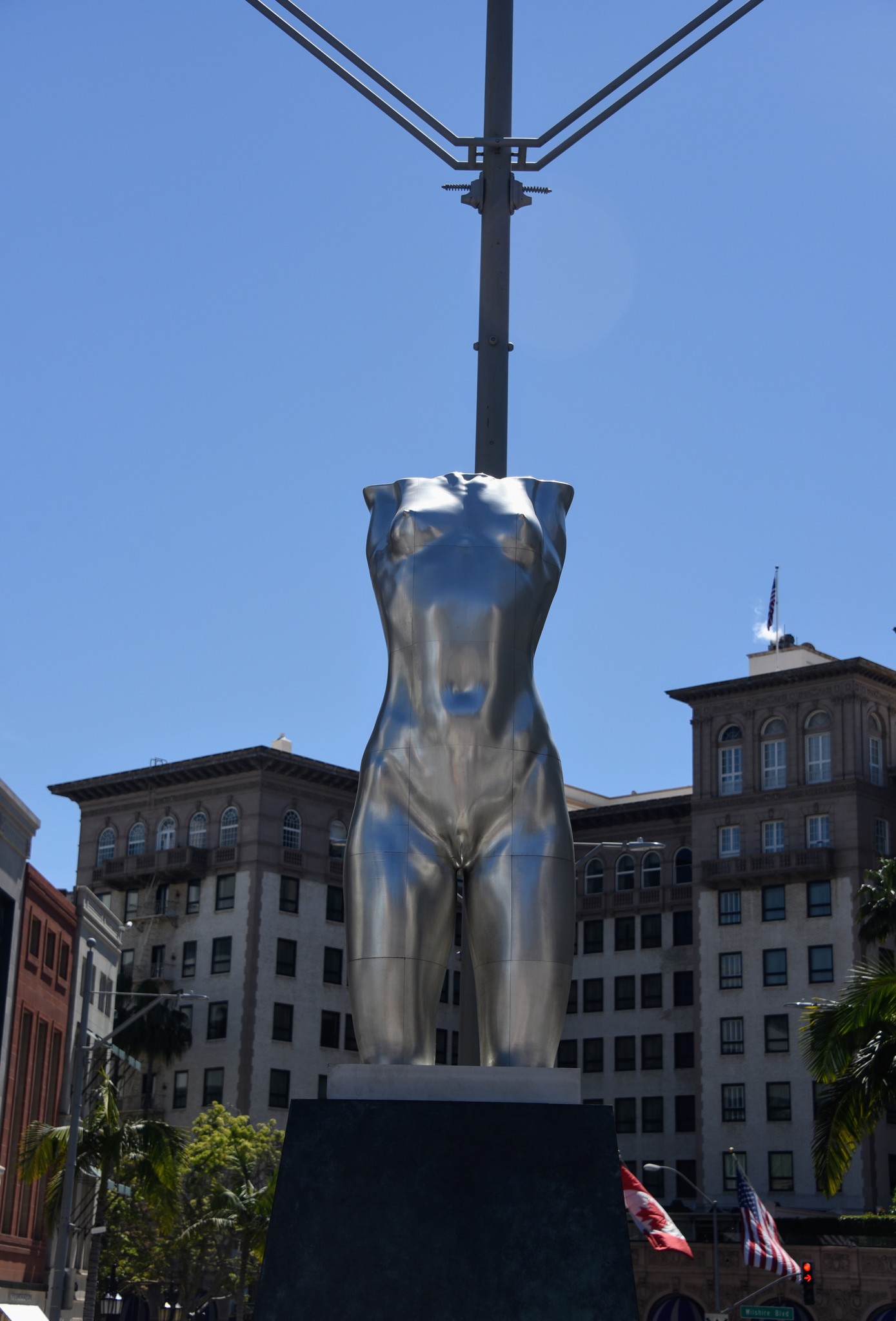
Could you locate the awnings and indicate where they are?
[0,1304,49,1321]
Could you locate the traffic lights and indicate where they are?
[802,1262,816,1305]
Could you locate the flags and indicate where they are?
[767,572,776,631]
[620,1154,694,1260]
[732,1153,802,1285]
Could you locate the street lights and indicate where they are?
[45,937,211,1321]
[643,1163,719,1321]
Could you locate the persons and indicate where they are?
[343,470,575,1069]
